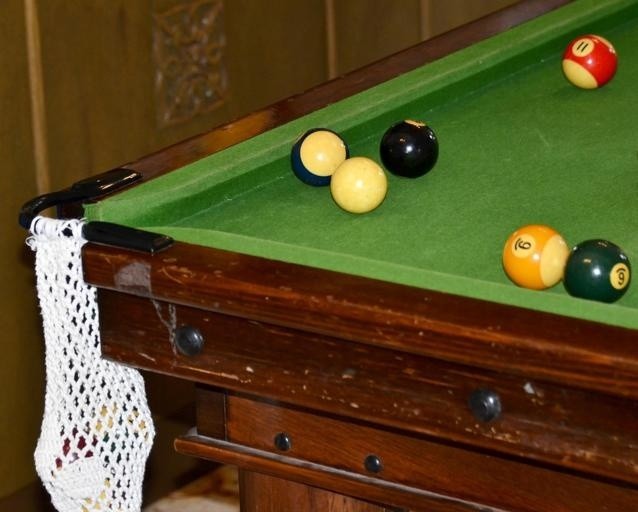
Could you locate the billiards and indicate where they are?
[290,127,350,188]
[331,155,388,214]
[500,226,571,291]
[561,33,618,91]
[39,398,150,512]
[379,119,440,180]
[564,238,632,304]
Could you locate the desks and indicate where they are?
[15,2,637,511]
